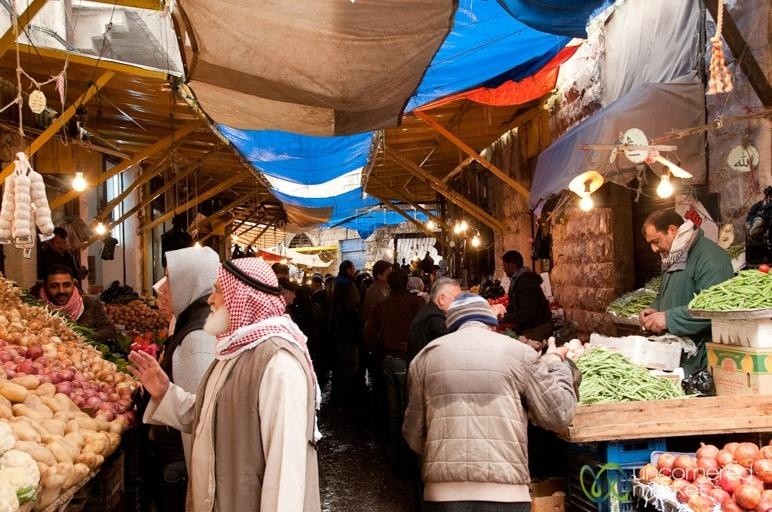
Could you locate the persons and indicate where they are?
[125,256,324,511]
[28,262,117,347]
[391,277,463,509]
[270,257,461,414]
[500,249,554,343]
[401,290,578,511]
[130,244,221,510]
[36,227,87,290]
[637,209,739,377]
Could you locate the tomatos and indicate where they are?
[759,264,769,272]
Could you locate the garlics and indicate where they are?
[0,170,54,242]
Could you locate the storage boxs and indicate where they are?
[705,318,772,396]
[527,477,565,512]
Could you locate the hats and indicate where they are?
[445,290,499,333]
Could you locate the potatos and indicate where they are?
[0,368,124,512]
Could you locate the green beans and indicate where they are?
[688,269,772,311]
[573,347,700,405]
[607,280,661,316]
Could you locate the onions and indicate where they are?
[639,439,772,512]
[0,340,136,425]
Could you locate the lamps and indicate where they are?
[657,165,674,197]
[71,163,88,193]
[93,217,106,235]
[578,182,594,212]
[453,212,480,247]
